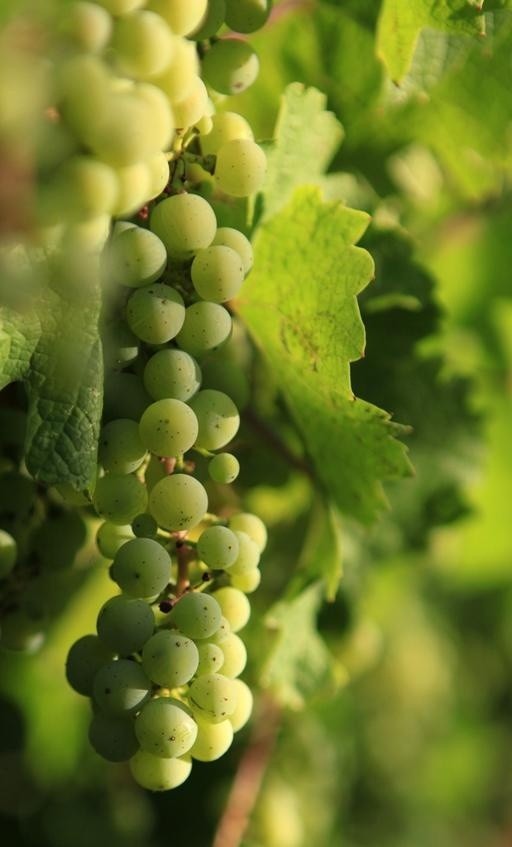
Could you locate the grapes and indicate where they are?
[0,0,274,790]
